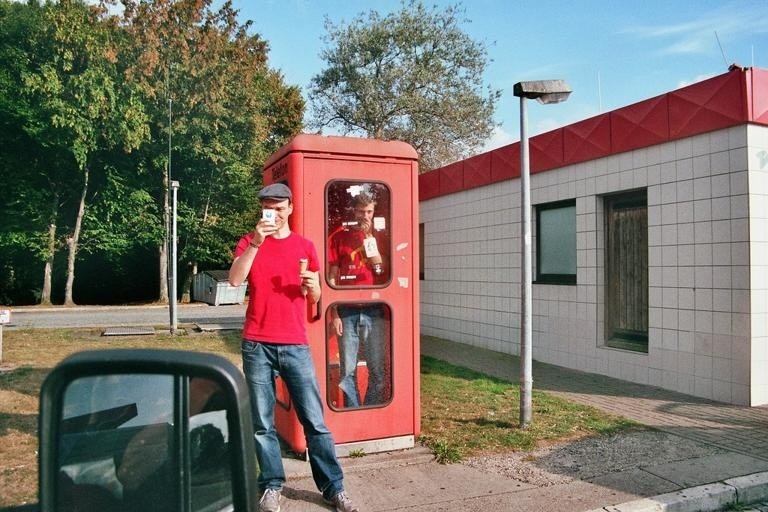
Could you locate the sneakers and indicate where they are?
[259,489,280,512]
[323,491,360,512]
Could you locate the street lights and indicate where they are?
[512,77,572,431]
[170,178,180,335]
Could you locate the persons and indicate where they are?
[88,372,230,511]
[228,183,356,512]
[327,182,392,409]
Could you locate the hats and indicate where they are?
[258,183,290,204]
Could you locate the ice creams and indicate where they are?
[299,259,308,274]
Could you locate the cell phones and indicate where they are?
[262,209,275,223]
